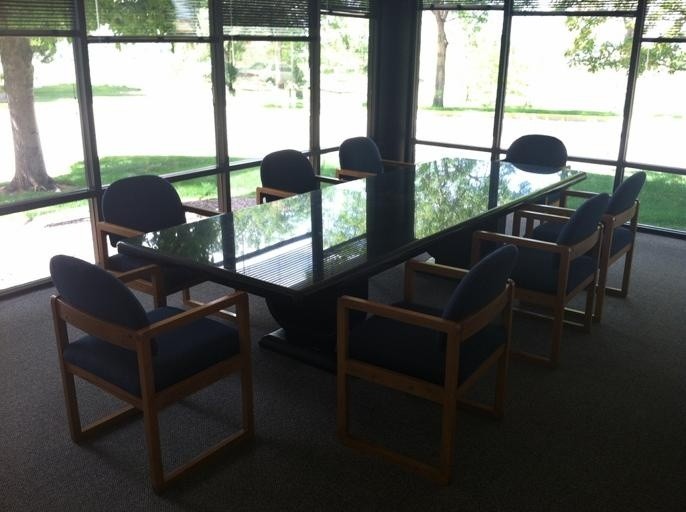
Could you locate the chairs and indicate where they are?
[473,191,611,367]
[255,148,350,205]
[503,134,568,171]
[335,245,518,483]
[48,249,254,492]
[335,136,415,177]
[524,168,647,330]
[96,174,232,310]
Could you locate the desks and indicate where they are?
[116,156,589,361]
